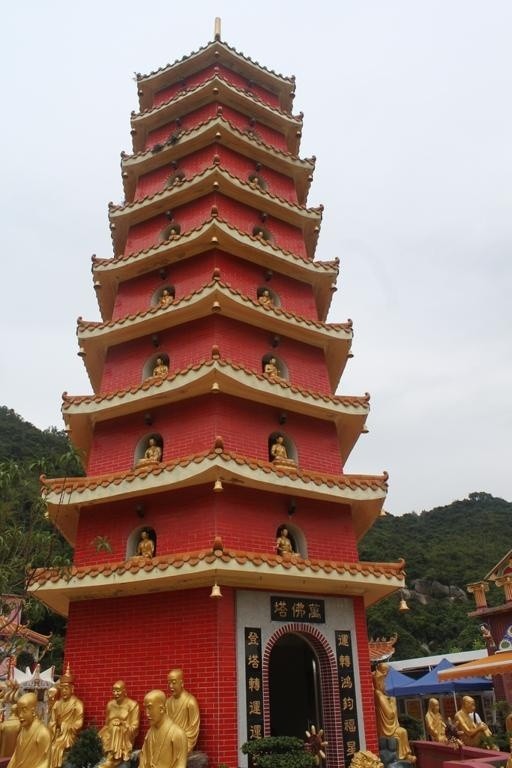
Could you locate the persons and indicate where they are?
[158,289,173,306]
[138,529,155,561]
[169,229,181,242]
[258,231,264,241]
[154,358,169,379]
[480,624,498,655]
[145,438,162,462]
[372,661,512,768]
[259,290,271,305]
[1,659,200,767]
[271,433,293,462]
[275,527,293,554]
[174,176,180,185]
[251,177,260,185]
[265,356,278,378]
[249,132,253,136]
